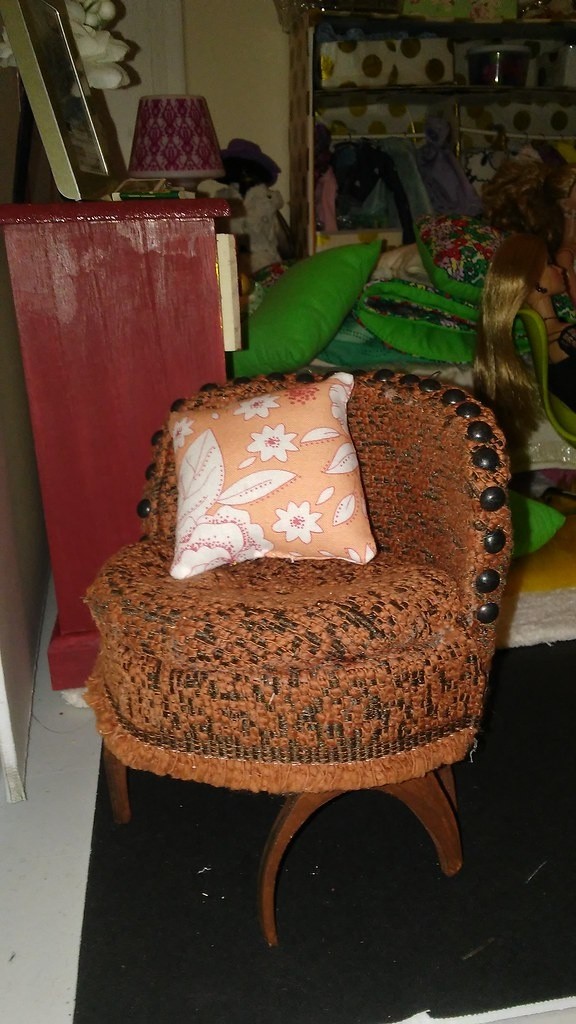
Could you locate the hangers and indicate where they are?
[348,131,565,150]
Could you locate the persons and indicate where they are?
[473,186,576,430]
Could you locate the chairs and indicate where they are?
[85,376,522,940]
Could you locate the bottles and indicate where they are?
[559,40,576,88]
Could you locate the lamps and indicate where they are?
[127,97,224,181]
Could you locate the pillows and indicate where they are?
[168,370,379,582]
[229,236,384,378]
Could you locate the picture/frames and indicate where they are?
[0,0,117,200]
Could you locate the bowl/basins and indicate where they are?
[465,45,531,87]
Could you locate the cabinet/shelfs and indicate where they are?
[1,201,242,693]
[286,9,576,260]
